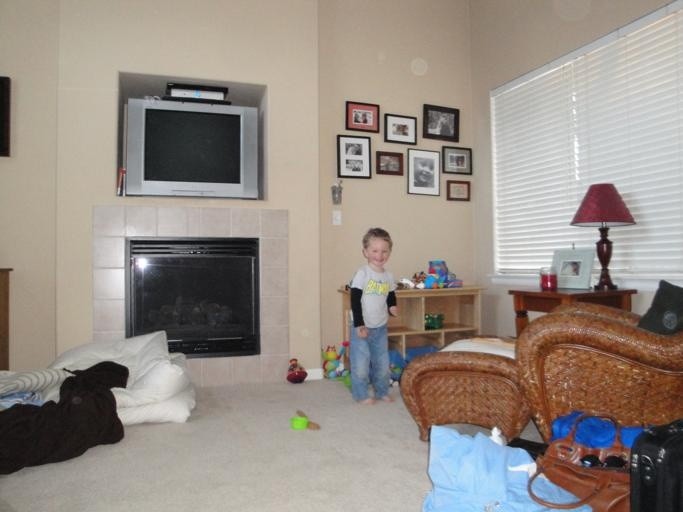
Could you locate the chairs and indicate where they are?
[515,301,682,446]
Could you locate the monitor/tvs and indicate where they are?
[125,236,259,358]
[125,97,260,200]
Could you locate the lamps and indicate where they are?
[570,183,636,292]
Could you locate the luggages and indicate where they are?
[630,419,683,511]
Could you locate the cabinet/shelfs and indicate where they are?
[337,286,487,372]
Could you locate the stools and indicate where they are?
[399,332,529,444]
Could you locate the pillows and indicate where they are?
[637,280,683,335]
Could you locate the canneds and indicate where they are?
[540,267,557,290]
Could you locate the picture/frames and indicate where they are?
[336,101,473,202]
[551,247,595,289]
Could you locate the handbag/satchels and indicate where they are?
[536,439,630,512]
[551,409,642,449]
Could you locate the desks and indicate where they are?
[508,285,637,340]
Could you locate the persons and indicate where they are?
[349,227,400,407]
[415,158,433,187]
